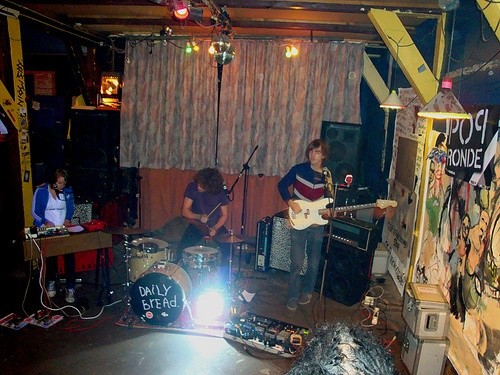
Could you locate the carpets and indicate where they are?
[117,269,254,337]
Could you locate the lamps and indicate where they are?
[214,44,234,65]
[212,34,230,53]
[168,0,204,23]
[416,0,471,119]
[379,46,407,109]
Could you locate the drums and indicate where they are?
[129,261,193,327]
[180,246,223,282]
[127,237,174,284]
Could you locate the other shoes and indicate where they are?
[47,280,56,297]
[287,296,298,310]
[298,291,312,304]
[64,285,76,303]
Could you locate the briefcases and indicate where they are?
[371,242,390,275]
[255,216,273,272]
[401,288,450,339]
[401,324,451,375]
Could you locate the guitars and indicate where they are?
[287,197,399,231]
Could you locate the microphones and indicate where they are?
[322,167,329,188]
[221,182,229,198]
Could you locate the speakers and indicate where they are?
[313,235,375,306]
[320,121,362,184]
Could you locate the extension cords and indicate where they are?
[371,307,379,325]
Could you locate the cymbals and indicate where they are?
[211,233,250,244]
[100,226,155,235]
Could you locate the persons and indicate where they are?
[278,139,337,312]
[32,168,76,303]
[176,168,236,282]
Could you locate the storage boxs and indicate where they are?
[371,243,389,274]
[400,282,451,375]
